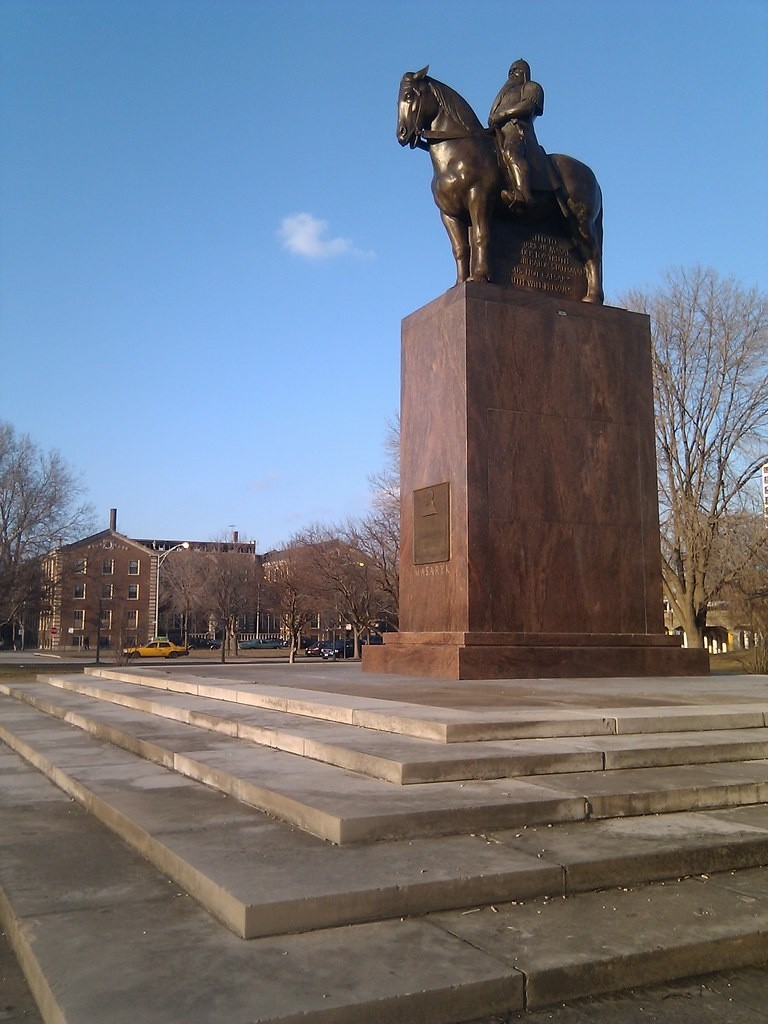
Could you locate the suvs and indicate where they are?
[321,638,366,659]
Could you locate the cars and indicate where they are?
[305,641,334,657]
[189,637,315,654]
[361,634,384,646]
[123,642,188,659]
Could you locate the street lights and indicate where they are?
[155,542,190,640]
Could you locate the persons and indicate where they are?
[488,58,544,209]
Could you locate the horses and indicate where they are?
[397,64,604,305]
